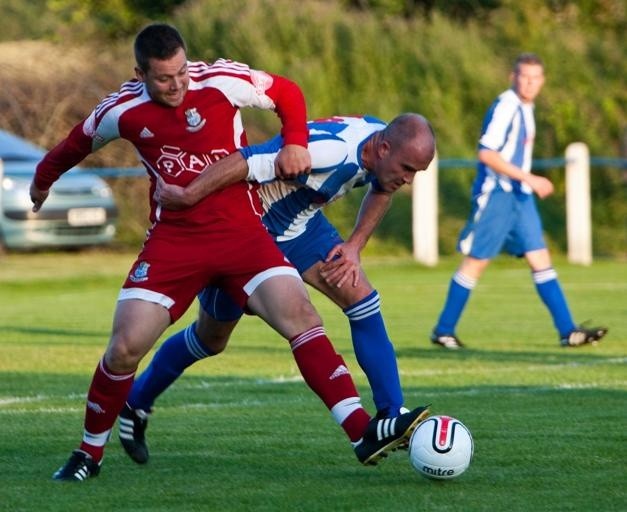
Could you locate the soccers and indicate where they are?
[408,415,474,481]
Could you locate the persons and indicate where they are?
[429,53,608,350]
[28,23,430,482]
[118,112,436,466]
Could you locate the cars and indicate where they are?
[1,126,118,251]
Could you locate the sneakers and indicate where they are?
[119,400,152,463]
[51,448,104,482]
[350,406,430,468]
[560,320,607,347]
[432,332,462,349]
